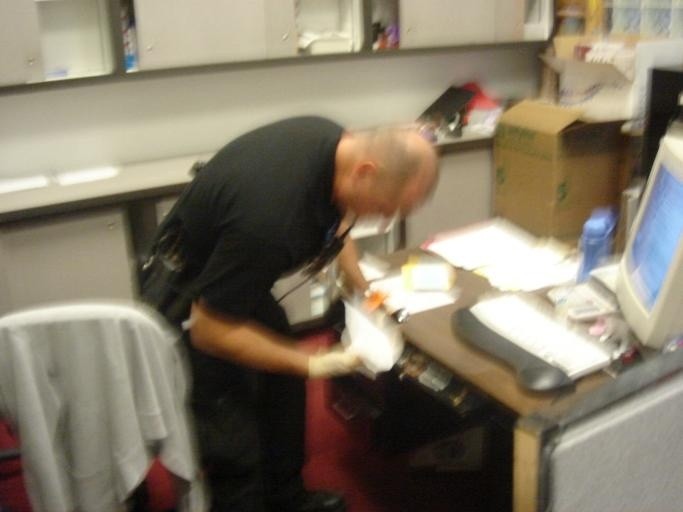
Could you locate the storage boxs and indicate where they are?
[534,30,664,96]
[489,96,639,254]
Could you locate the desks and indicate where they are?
[340,207,681,512]
[0,104,496,315]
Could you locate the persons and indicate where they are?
[136,116,444,511]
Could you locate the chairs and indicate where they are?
[0,304,194,510]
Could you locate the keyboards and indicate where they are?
[452,294,612,392]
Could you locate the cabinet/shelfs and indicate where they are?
[0,1,559,96]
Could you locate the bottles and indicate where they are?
[580,216,611,276]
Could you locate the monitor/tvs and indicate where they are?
[613,134,683,354]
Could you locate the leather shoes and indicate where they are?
[301,490,347,511]
